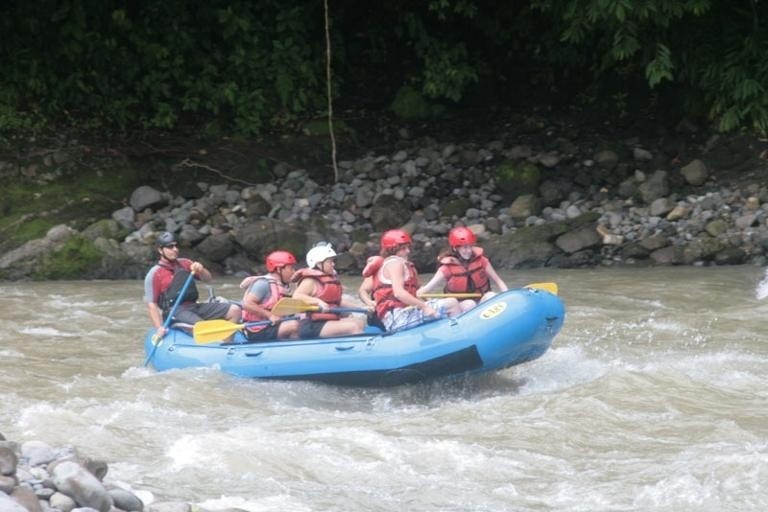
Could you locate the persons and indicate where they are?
[144,229,242,343]
[239,248,300,341]
[289,244,366,338]
[356,230,465,331]
[414,225,509,320]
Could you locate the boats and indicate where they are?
[143,286,565,386]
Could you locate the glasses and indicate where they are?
[165,243,179,249]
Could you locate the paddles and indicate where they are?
[194,314,301,345]
[420,282,560,298]
[271,298,379,315]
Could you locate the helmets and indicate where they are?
[448,227,476,246]
[381,230,410,248]
[306,246,336,269]
[156,231,178,248]
[265,251,297,274]
